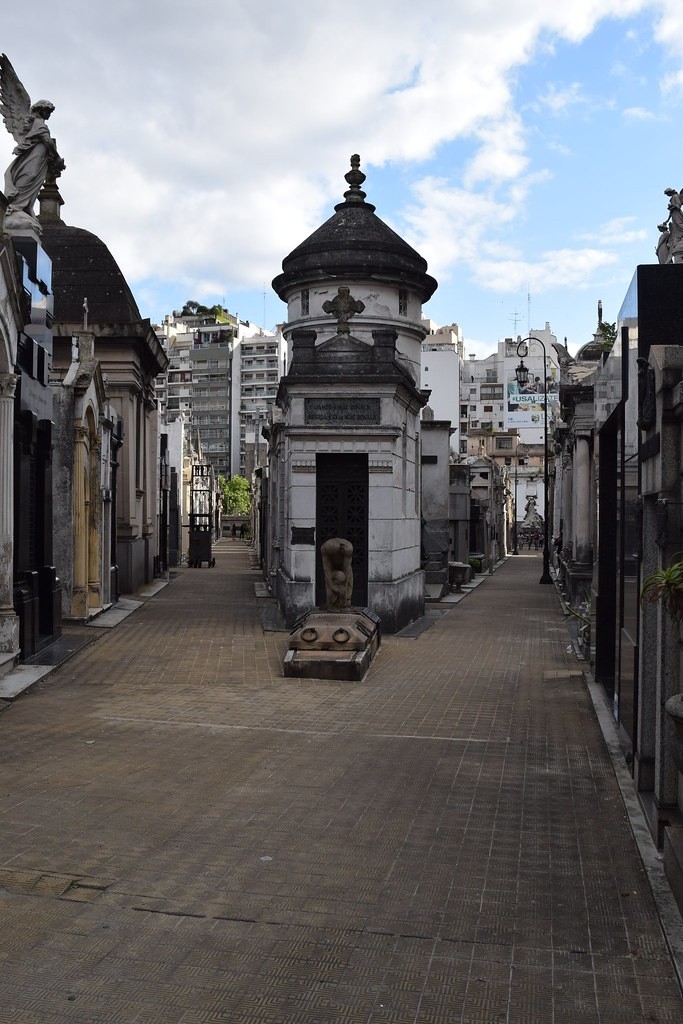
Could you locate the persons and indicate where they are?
[555,531,563,567]
[515,376,558,393]
[662,188,683,242]
[655,224,672,264]
[517,531,544,550]
[4,100,66,215]
[231,524,245,540]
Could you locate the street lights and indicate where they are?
[516,337,554,585]
